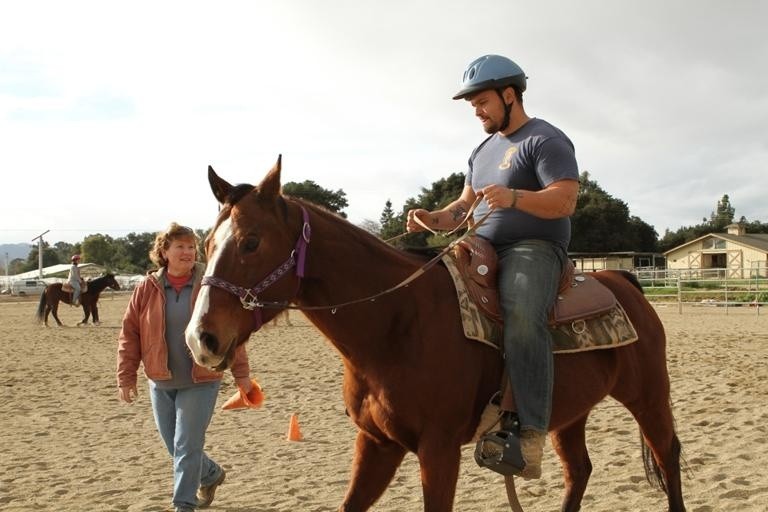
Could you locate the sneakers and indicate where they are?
[197,470,226,509]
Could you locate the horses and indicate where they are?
[183,153,681,512]
[37,273,120,329]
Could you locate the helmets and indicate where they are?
[72,255,80,260]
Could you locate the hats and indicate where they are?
[452,54,527,101]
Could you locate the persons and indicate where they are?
[69,255,84,305]
[116,219,254,512]
[405,54,583,478]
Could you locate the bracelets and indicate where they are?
[510,189,519,208]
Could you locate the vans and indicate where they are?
[11,279,48,296]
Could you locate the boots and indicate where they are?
[475,412,545,480]
